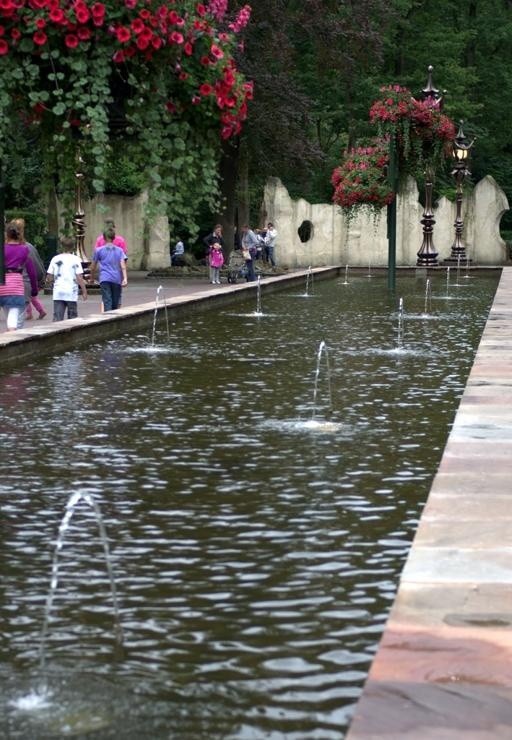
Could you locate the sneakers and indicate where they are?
[37,310,47,319]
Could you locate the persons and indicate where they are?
[11,218,47,322]
[239,223,260,282]
[0,222,40,331]
[264,222,277,268]
[209,243,224,285]
[203,223,224,283]
[88,228,130,312]
[44,237,88,323]
[170,234,184,261]
[234,225,269,262]
[94,215,128,311]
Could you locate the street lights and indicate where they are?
[439,119,477,266]
[415,63,448,267]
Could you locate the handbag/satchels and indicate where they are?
[243,250,252,261]
